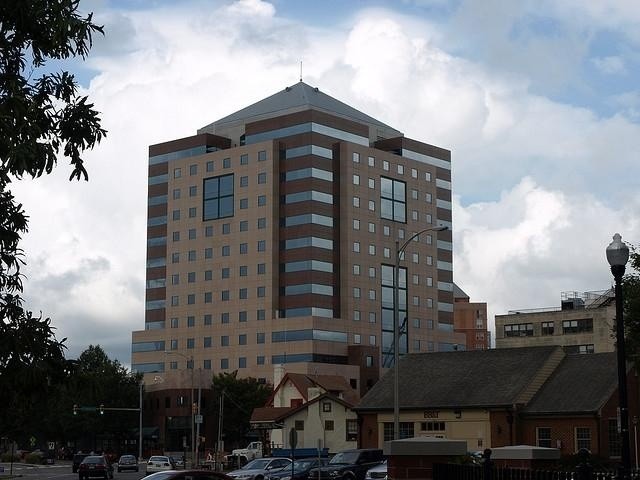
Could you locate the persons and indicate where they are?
[479,448,494,466]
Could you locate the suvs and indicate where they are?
[309,449,383,480]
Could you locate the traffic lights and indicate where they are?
[101,412,103,414]
[74,412,76,415]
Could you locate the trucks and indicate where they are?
[232,442,329,466]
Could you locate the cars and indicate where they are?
[72,453,114,480]
[139,470,234,480]
[365,460,387,480]
[226,457,293,480]
[117,455,139,473]
[264,459,328,479]
[146,456,176,474]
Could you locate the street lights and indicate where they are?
[164,351,195,464]
[394,226,449,440]
[606,233,630,477]
